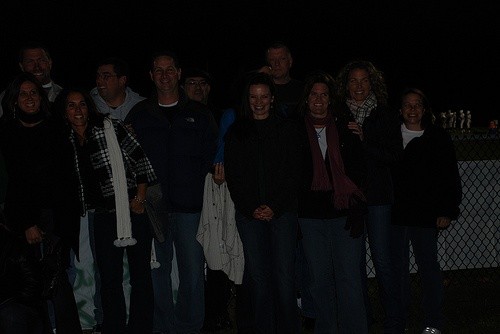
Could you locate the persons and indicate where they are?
[460,110,465,135]
[467,109,472,131]
[449,111,453,130]
[0,48,459,333]
[440,111,447,131]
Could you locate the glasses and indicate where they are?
[184,81,208,87]
[96,74,114,80]
[403,103,423,108]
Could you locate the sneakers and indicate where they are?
[423,327,441,334]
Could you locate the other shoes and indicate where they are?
[385,331,397,334]
[210,321,235,334]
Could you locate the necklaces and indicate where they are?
[316,126,326,141]
